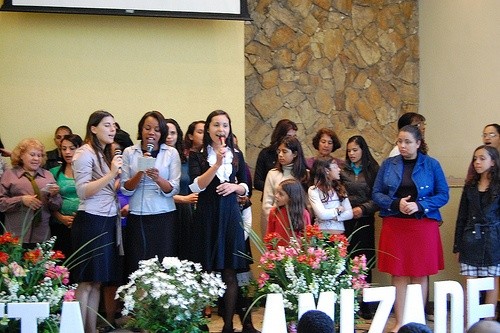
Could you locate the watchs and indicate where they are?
[336,207,342,216]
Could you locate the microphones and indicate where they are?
[115,147,122,174]
[218,134,226,158]
[147,141,154,153]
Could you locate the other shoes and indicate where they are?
[204,313,212,322]
[222,324,262,333]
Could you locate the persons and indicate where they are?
[466,124,500,185]
[453,145,500,322]
[266,180,311,252]
[308,157,352,244]
[467,320,500,333]
[0,109,450,333]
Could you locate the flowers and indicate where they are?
[0,202,114,333]
[115,255,227,333]
[236,204,400,333]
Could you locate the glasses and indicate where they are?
[481,133,499,137]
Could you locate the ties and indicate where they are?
[24,173,42,227]
